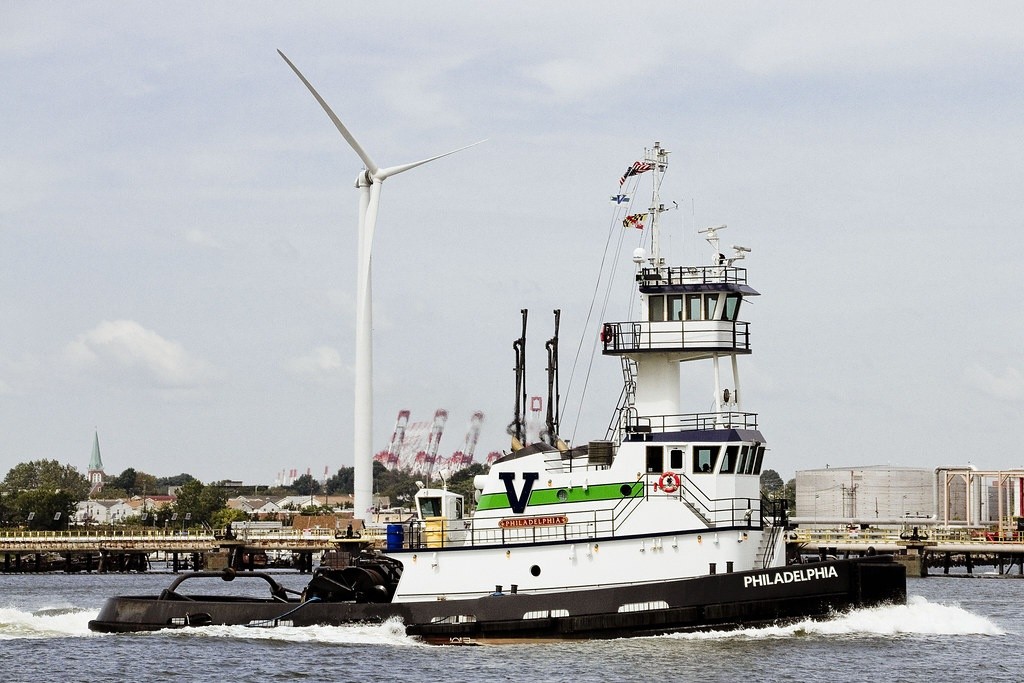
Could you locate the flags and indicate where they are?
[623,212,648,231]
[620,161,650,187]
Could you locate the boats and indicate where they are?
[391,139,907,645]
[88,538,404,633]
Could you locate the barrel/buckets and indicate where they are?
[588,441,613,465]
[386,525,404,551]
[425,516,447,548]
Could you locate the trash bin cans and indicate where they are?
[425,516,450,549]
